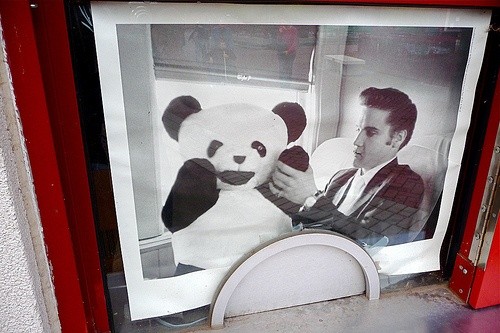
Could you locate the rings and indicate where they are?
[275,190,282,199]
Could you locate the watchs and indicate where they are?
[299,189,323,213]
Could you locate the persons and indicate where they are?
[267,86,425,248]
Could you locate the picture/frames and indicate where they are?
[73,0,494,322]
